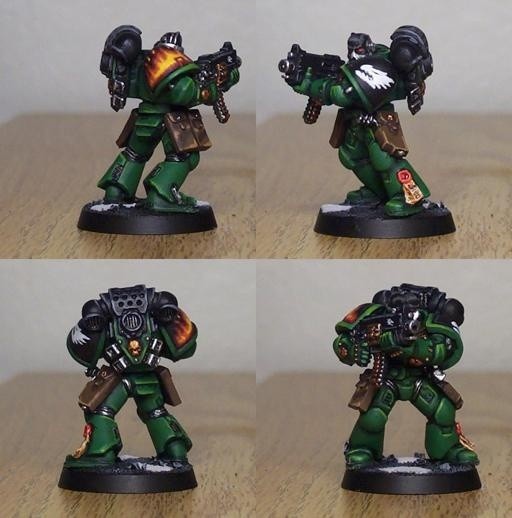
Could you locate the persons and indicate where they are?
[78,25,242,236]
[279,23,456,239]
[334,281,482,497]
[57,284,202,495]
